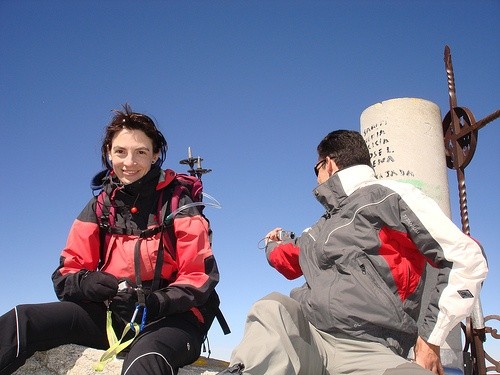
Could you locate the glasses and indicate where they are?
[314,156,335,176]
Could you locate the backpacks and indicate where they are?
[96,171,231,336]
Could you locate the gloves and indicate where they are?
[81,270,119,303]
[114,288,161,324]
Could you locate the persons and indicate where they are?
[0,103,221,375]
[216,130,488,375]
